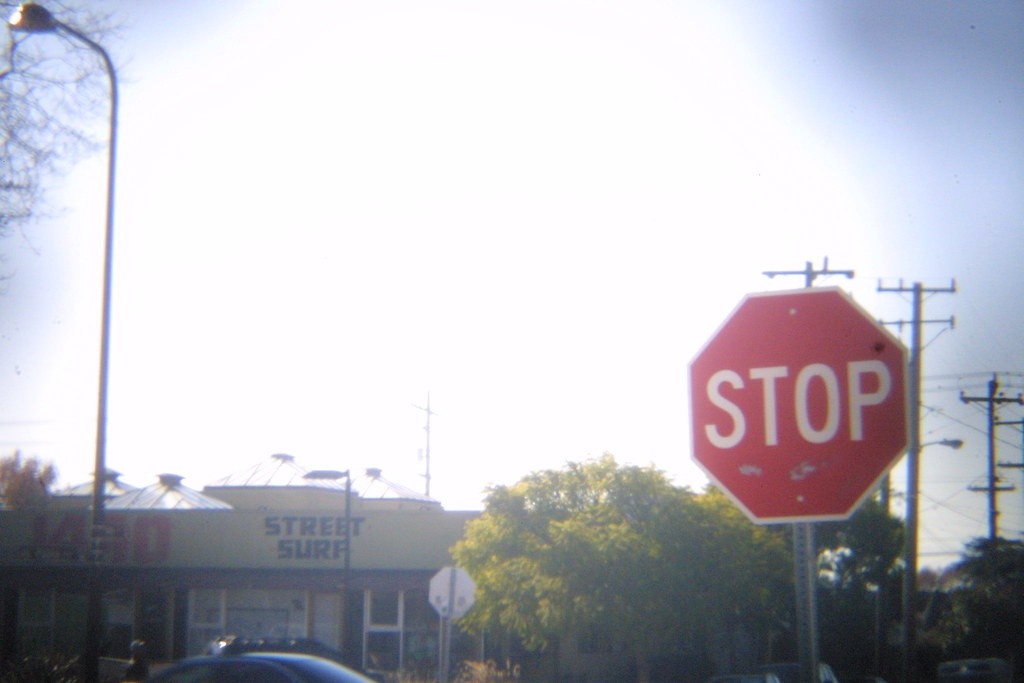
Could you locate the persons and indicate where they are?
[125,640,149,683]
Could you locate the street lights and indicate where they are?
[897,435,967,683]
[7,1,122,683]
[296,468,356,666]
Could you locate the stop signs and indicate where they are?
[685,285,915,526]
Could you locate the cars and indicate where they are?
[938,658,1014,683]
[706,660,888,683]
[140,633,382,683]
